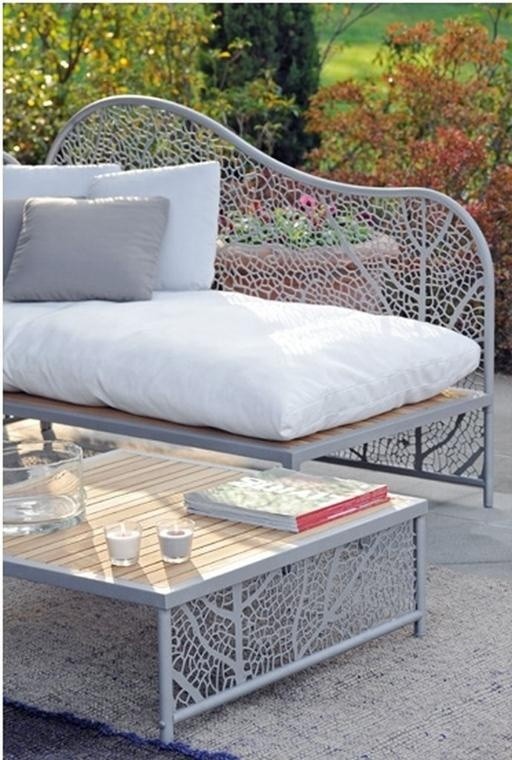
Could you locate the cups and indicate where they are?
[104,519,195,565]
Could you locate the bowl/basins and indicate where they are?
[4,439,87,537]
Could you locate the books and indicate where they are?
[182,465,391,535]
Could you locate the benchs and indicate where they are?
[3,94,497,576]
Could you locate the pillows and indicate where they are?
[89,158,219,294]
[4,162,121,281]
[2,290,483,442]
[4,196,173,303]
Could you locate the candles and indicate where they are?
[154,522,194,560]
[103,520,141,561]
